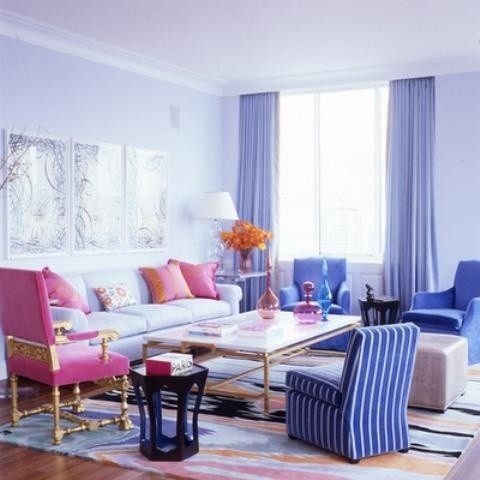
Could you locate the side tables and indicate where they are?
[129,362,209,462]
[215,272,267,312]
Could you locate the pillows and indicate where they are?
[42,259,220,313]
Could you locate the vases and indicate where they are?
[239,254,253,273]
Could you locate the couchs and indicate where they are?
[42,266,243,362]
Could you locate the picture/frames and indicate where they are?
[4,127,169,260]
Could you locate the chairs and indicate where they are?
[0,267,134,445]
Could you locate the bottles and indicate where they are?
[256,255,281,320]
[316,256,332,323]
[292,281,323,324]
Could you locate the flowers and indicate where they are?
[221,221,274,259]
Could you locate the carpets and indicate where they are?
[0,347,480,480]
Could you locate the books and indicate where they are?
[237,319,283,338]
[187,320,236,337]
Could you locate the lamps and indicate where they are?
[195,192,240,271]
